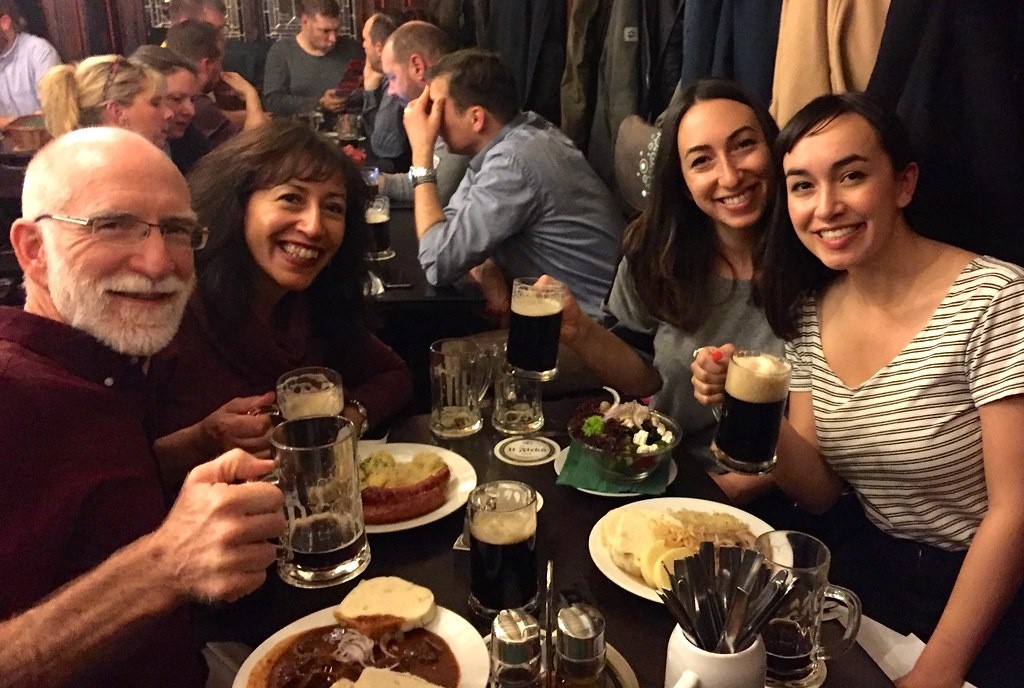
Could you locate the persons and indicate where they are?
[525,78,781,533]
[403,48,627,334]
[180,117,410,441]
[0,128,283,688]
[0,0,471,205]
[688,89,1024,688]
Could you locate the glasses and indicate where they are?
[34,215,209,250]
[102,54,133,102]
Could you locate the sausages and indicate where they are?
[358,465,450,524]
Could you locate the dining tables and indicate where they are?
[200,392,896,688]
[357,206,486,413]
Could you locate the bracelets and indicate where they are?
[413,175,438,187]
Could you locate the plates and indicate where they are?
[553,445,678,497]
[357,442,477,533]
[232,604,490,688]
[588,497,794,612]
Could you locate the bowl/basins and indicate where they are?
[567,408,683,485]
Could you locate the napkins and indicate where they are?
[200,641,255,688]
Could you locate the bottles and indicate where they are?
[489,608,543,688]
[554,603,607,688]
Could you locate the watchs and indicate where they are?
[349,400,369,439]
[408,166,436,182]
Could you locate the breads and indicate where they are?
[326,665,444,688]
[602,508,694,592]
[333,576,435,640]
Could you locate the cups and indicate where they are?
[360,166,379,196]
[427,337,492,439]
[247,367,346,421]
[753,530,863,688]
[709,347,793,478]
[292,111,362,150]
[363,195,392,258]
[467,480,543,620]
[246,414,372,589]
[664,622,766,688]
[490,341,544,436]
[501,277,567,382]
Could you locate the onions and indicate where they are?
[602,386,643,431]
[325,626,402,671]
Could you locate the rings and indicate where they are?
[692,347,704,358]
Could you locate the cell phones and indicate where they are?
[377,269,414,288]
